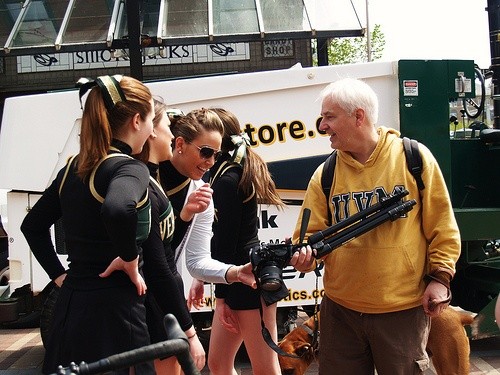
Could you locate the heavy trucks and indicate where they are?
[2,56,499,344]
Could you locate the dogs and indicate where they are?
[276,299,480,375]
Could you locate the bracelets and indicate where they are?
[188,334,195,339]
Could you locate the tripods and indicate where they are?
[283,189,415,261]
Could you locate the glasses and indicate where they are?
[185,140,224,163]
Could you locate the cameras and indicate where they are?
[250,239,295,293]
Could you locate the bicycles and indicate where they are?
[50,313,202,375]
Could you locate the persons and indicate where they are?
[158,107,262,294]
[187,109,290,375]
[130,98,207,375]
[285,78,462,375]
[20,75,154,375]
